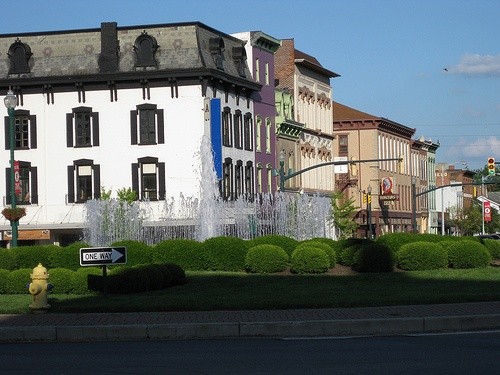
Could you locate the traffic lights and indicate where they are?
[487,157,495,176]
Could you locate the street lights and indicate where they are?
[411,176,417,232]
[367,184,373,240]
[441,160,467,236]
[3,85,19,249]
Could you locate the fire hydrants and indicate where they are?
[27,261,54,314]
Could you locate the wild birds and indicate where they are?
[443,67,449,72]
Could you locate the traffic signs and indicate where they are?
[79,246,128,267]
